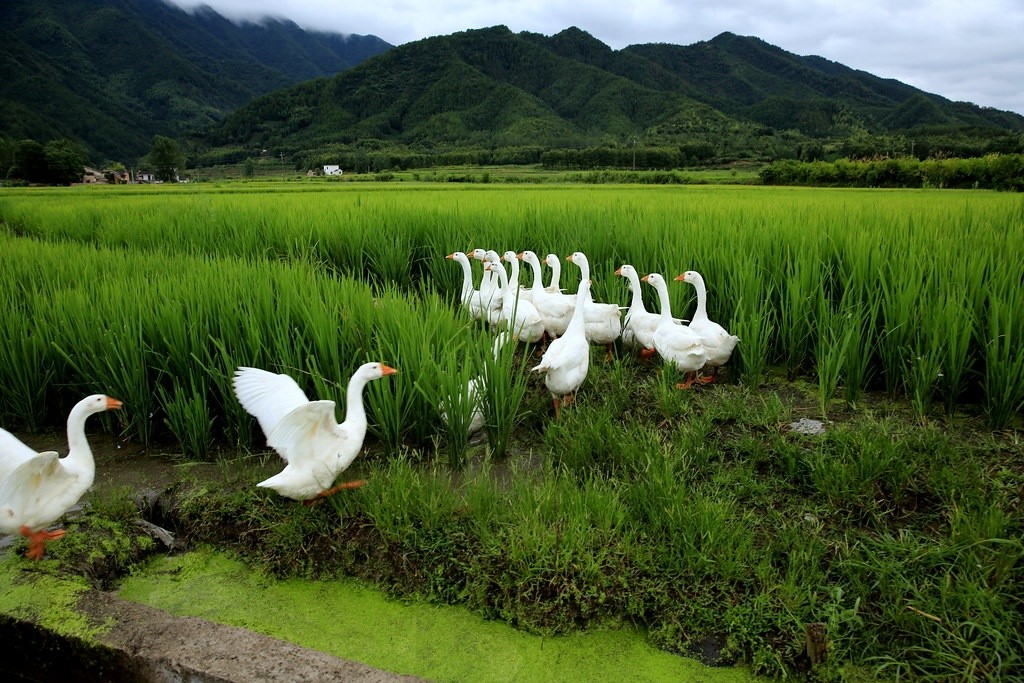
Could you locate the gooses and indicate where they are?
[231,361,398,505]
[438,248,742,438]
[0,394,123,562]
